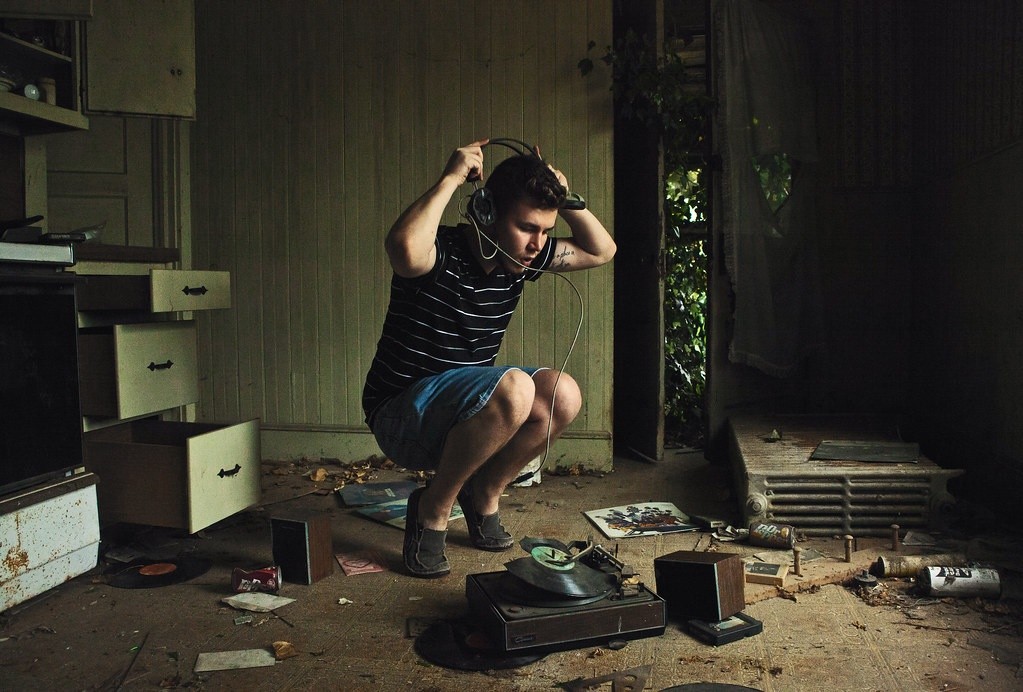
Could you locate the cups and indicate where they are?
[37,78,56,106]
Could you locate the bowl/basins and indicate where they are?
[1,64,23,91]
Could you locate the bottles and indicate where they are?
[916,566,1002,598]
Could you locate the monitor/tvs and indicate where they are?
[0,262,85,497]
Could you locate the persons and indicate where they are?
[361,137,617,577]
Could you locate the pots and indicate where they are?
[42,219,108,244]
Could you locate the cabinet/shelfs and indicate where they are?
[0,0,263,532]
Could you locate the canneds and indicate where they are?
[748,522,798,549]
[922,566,1003,599]
[231,566,282,591]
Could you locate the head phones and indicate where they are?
[458,136,586,225]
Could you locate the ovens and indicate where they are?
[0,267,86,495]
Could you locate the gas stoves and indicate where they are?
[0,229,76,267]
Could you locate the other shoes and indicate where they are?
[402,486,451,578]
[457,473,515,551]
